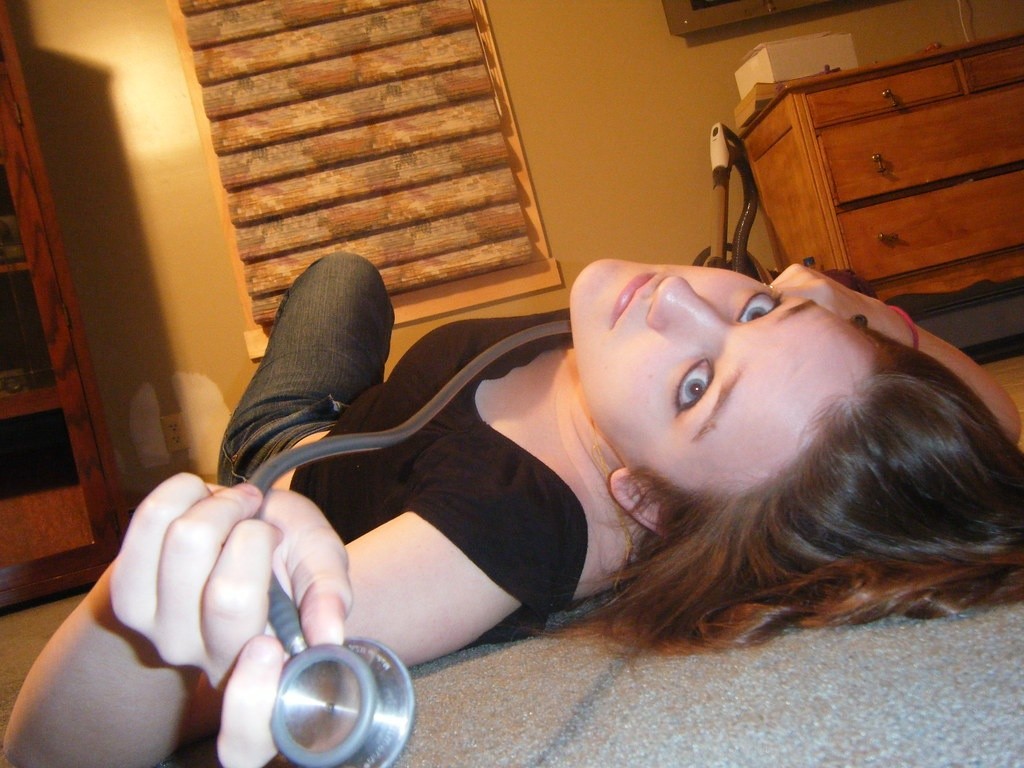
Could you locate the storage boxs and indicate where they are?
[733,30,856,101]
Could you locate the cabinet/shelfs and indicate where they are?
[661,0,828,36]
[740,30,1024,322]
[1,1,134,613]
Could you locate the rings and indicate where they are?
[769,286,773,288]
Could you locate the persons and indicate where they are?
[1,252,1023,768]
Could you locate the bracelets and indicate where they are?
[888,306,919,350]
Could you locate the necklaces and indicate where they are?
[590,418,635,586]
